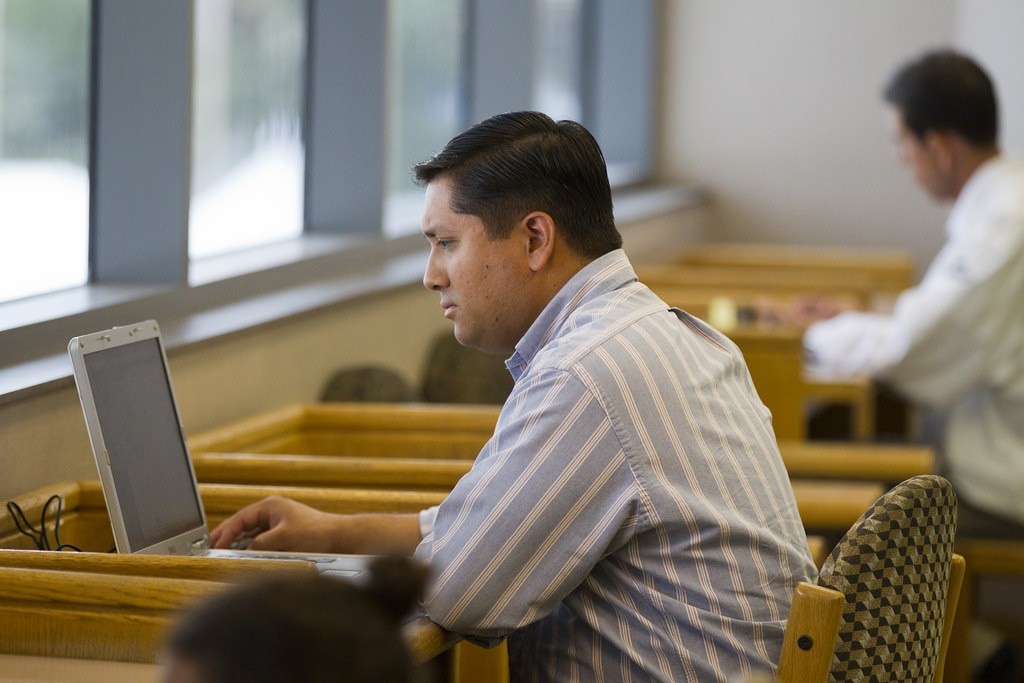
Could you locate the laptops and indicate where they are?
[69,319,401,592]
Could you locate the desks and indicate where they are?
[0,247,937,683]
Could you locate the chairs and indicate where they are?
[775,475,969,683]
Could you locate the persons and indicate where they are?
[790,51,1024,541]
[210,109,819,683]
[165,554,430,683]
